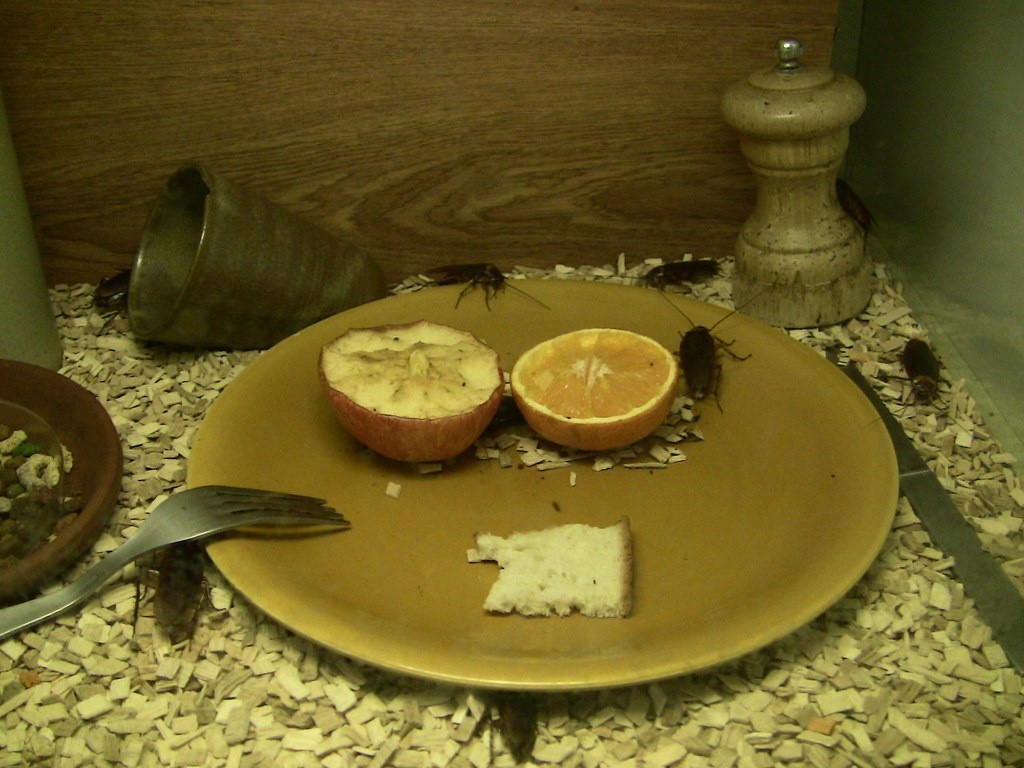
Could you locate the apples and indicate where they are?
[318,320,506,462]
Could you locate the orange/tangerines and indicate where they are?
[508,328,678,450]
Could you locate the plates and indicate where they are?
[0,359,125,606]
[186,276,901,695]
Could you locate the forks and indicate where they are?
[0,484,351,644]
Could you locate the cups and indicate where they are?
[129,166,389,352]
[0,95,62,373]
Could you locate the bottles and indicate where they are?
[721,39,873,327]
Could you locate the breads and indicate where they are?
[466,515,633,619]
